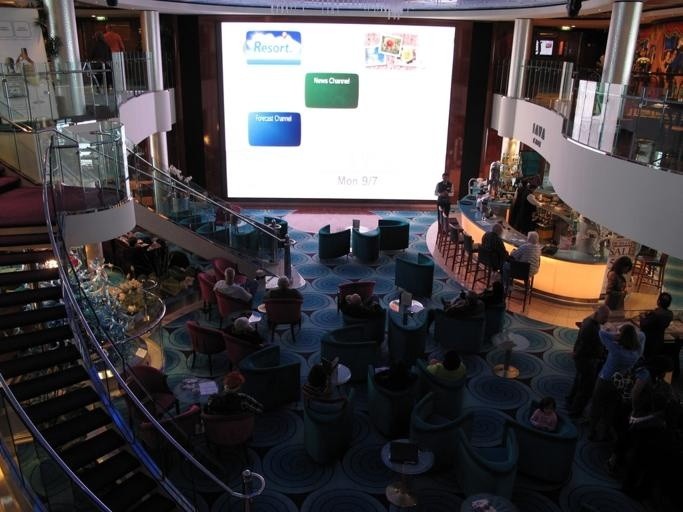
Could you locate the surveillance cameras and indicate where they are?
[106,0,117,6]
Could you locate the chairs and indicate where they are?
[118,205,682,477]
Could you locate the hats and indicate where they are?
[223,371,244,392]
[234,317,250,331]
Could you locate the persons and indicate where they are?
[202,371,265,419]
[263,275,304,335]
[510,231,541,277]
[90,23,127,95]
[663,37,683,94]
[435,172,452,223]
[529,396,558,432]
[339,292,386,321]
[563,255,683,497]
[223,317,267,352]
[301,365,343,401]
[481,224,509,275]
[420,281,506,334]
[212,267,252,322]
[426,350,466,387]
[508,171,543,236]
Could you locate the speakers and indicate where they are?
[564,29,599,69]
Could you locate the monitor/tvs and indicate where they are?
[533,37,555,60]
